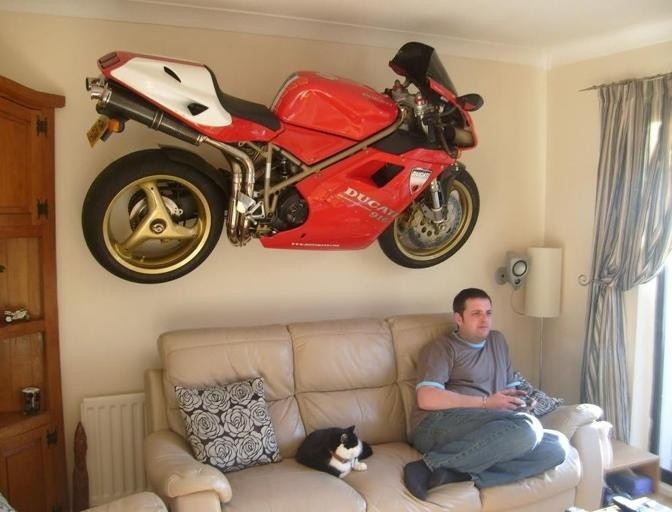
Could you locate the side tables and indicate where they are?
[603,438,659,499]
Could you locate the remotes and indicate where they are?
[613,495,651,512]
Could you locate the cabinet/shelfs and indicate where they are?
[0,71,77,512]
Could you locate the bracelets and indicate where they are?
[483,396,486,409]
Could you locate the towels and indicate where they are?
[72,421,90,512]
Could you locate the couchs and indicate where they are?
[138,367,612,512]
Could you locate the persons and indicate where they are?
[404,287,570,501]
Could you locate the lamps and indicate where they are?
[510,244,563,390]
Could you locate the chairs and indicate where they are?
[81,491,169,512]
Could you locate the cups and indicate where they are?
[21,386,41,416]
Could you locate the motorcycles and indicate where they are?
[76,40,486,287]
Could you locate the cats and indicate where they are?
[294,424,373,478]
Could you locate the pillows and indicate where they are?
[173,374,281,472]
[504,369,568,418]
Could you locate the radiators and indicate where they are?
[78,386,148,508]
